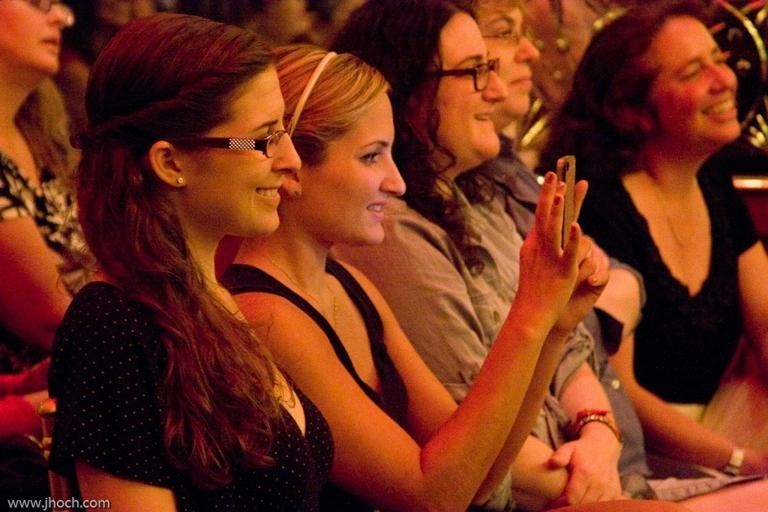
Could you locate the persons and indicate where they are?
[0,0,768,512]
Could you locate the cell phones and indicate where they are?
[556,155,576,249]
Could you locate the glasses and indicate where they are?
[425,57,502,94]
[477,24,527,47]
[159,125,292,158]
